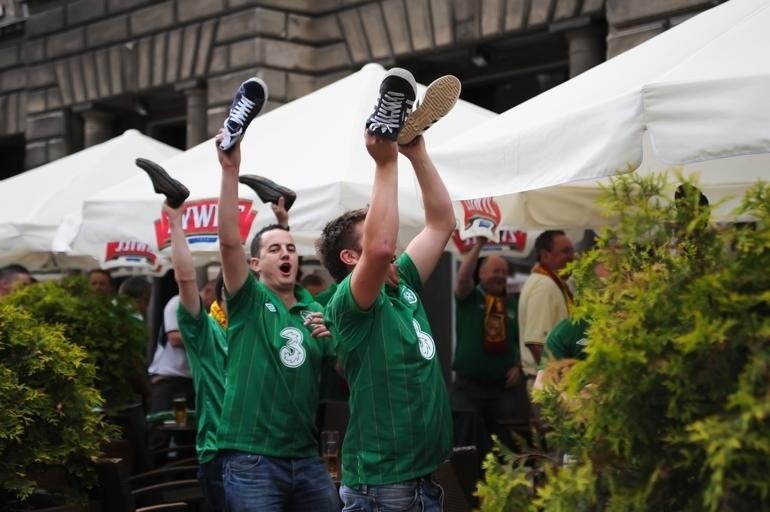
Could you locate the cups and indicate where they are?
[172,392,188,426]
[320,430,341,480]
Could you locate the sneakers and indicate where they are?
[136,157,190,209]
[219,76,267,152]
[367,68,416,141]
[398,75,462,147]
[239,175,296,212]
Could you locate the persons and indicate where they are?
[153,196,290,512]
[322,133,456,512]
[454,229,652,466]
[216,130,342,512]
[661,183,709,276]
[0,263,216,474]
